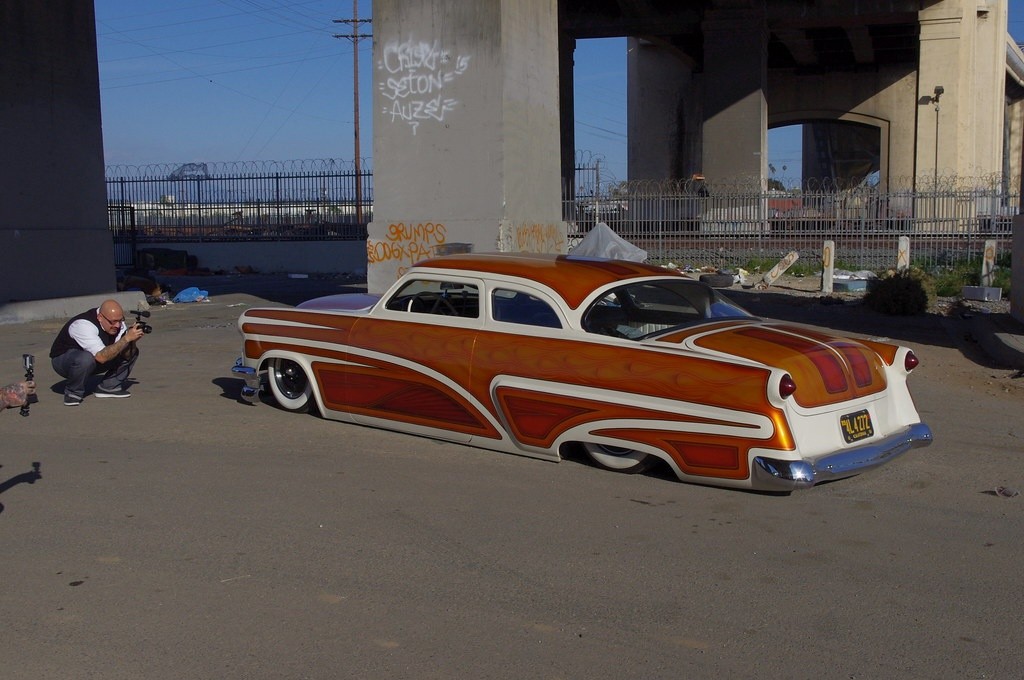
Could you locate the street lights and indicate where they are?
[931,85,945,219]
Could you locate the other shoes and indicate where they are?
[94,386,131,397]
[64,394,79,406]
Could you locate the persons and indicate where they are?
[0,379,37,412]
[49,300,144,406]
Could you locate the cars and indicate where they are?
[226,249,935,496]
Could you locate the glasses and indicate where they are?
[99,312,125,325]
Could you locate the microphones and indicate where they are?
[129,310,151,317]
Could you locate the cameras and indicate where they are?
[134,321,152,334]
